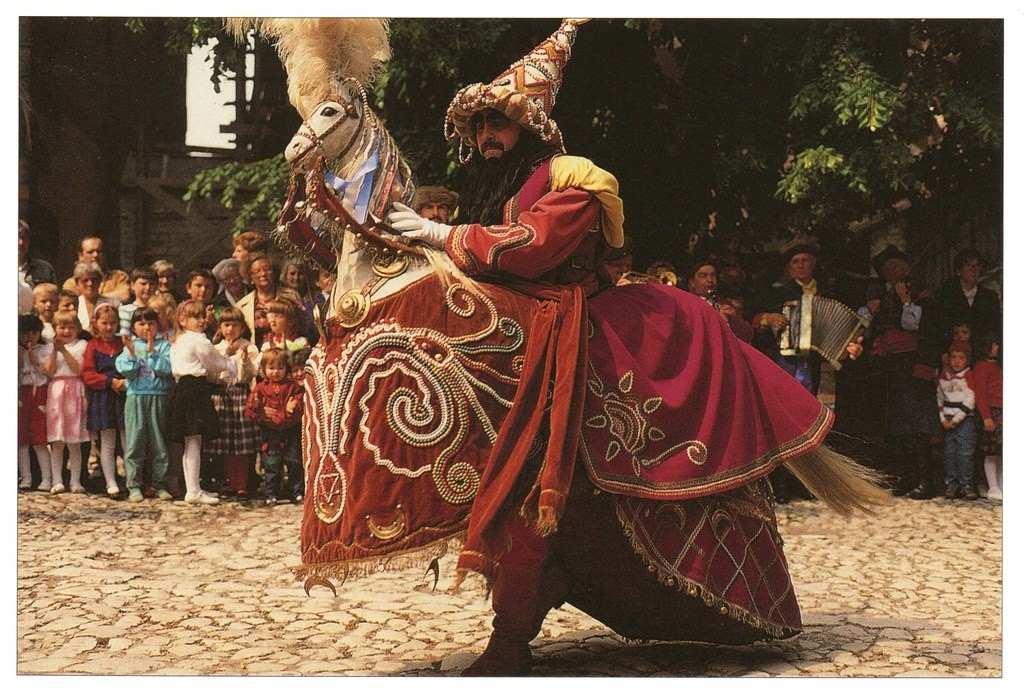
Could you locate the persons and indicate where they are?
[752,237,866,503]
[686,258,757,343]
[907,250,1003,499]
[412,183,461,221]
[35,232,337,507]
[597,235,679,287]
[19,219,57,315]
[390,82,838,678]
[866,246,934,497]
[19,314,50,490]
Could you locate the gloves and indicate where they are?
[386,200,456,250]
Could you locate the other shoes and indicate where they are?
[70,485,85,492]
[129,488,142,503]
[945,485,959,499]
[906,481,935,499]
[51,484,63,494]
[19,481,31,488]
[154,490,173,500]
[38,482,51,490]
[237,492,248,500]
[961,486,977,499]
[107,484,120,498]
[185,491,219,505]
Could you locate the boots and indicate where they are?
[527,558,570,642]
[459,610,536,676]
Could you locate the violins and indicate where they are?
[893,275,913,302]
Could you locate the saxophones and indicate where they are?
[708,287,729,327]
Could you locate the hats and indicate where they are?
[443,18,592,165]
[948,340,971,361]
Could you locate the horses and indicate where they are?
[281,76,897,575]
